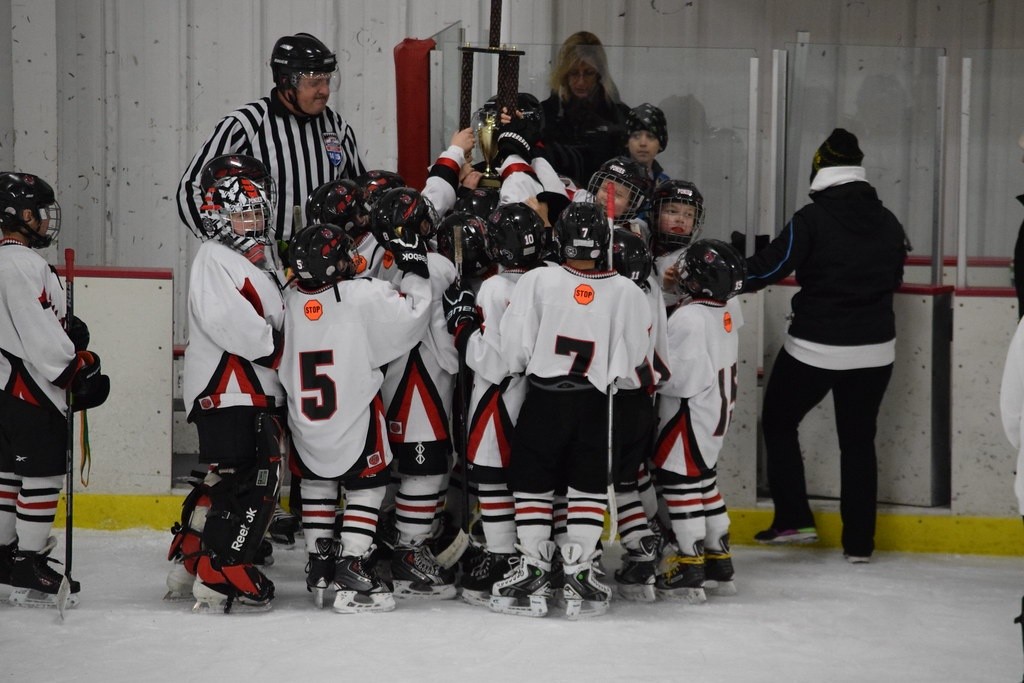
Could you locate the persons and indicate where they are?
[1000,217,1024,517]
[168,28,744,625]
[743,128,909,564]
[0,171,109,610]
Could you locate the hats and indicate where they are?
[623,103,669,153]
[812,127,866,171]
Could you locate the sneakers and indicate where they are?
[304,553,396,613]
[192,555,275,613]
[267,502,299,550]
[0,534,81,610]
[388,514,736,620]
[163,536,274,603]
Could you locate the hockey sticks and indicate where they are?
[62,247,82,594]
[435,225,472,571]
[223,205,303,616]
[605,181,619,546]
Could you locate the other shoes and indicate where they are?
[753,514,819,545]
[842,538,876,564]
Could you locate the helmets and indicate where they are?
[201,153,278,236]
[289,156,747,304]
[0,171,62,249]
[479,92,545,147]
[270,32,341,93]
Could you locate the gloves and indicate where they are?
[64,313,90,351]
[442,279,478,335]
[390,233,430,279]
[72,351,104,389]
